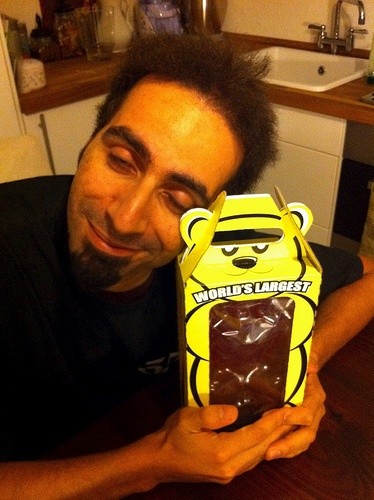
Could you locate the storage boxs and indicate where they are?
[13,58,47,94]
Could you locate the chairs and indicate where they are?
[0,134,54,184]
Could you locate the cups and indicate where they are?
[75,5,114,60]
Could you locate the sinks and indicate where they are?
[240,46,369,92]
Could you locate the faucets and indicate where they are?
[308,0,369,53]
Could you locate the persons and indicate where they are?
[0,32,374,500]
